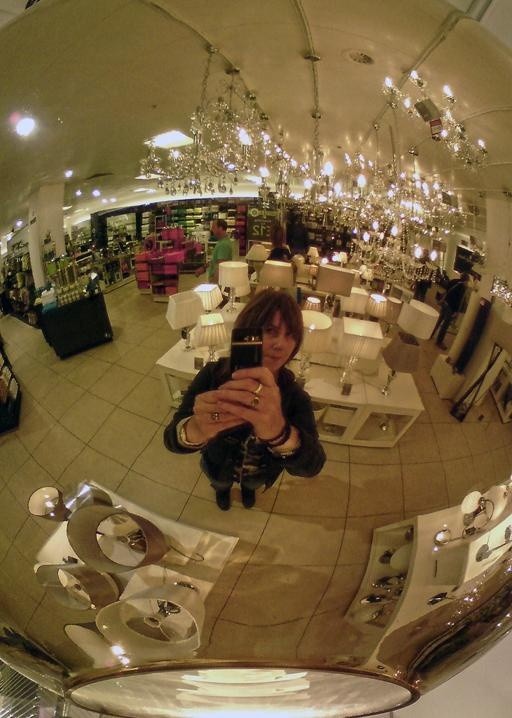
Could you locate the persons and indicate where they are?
[269,239,292,262]
[428,272,470,352]
[161,287,327,513]
[193,218,234,309]
[408,247,434,303]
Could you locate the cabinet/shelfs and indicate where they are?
[86,184,362,303]
[0,223,138,436]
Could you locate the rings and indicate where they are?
[212,411,221,423]
[253,383,263,395]
[251,396,260,409]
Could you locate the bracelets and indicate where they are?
[263,424,292,448]
[202,265,207,273]
[257,421,288,443]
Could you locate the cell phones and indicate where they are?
[230,328,263,369]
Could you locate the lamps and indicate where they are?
[342,477,512,671]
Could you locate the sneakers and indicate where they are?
[216,488,230,511]
[242,485,255,508]
